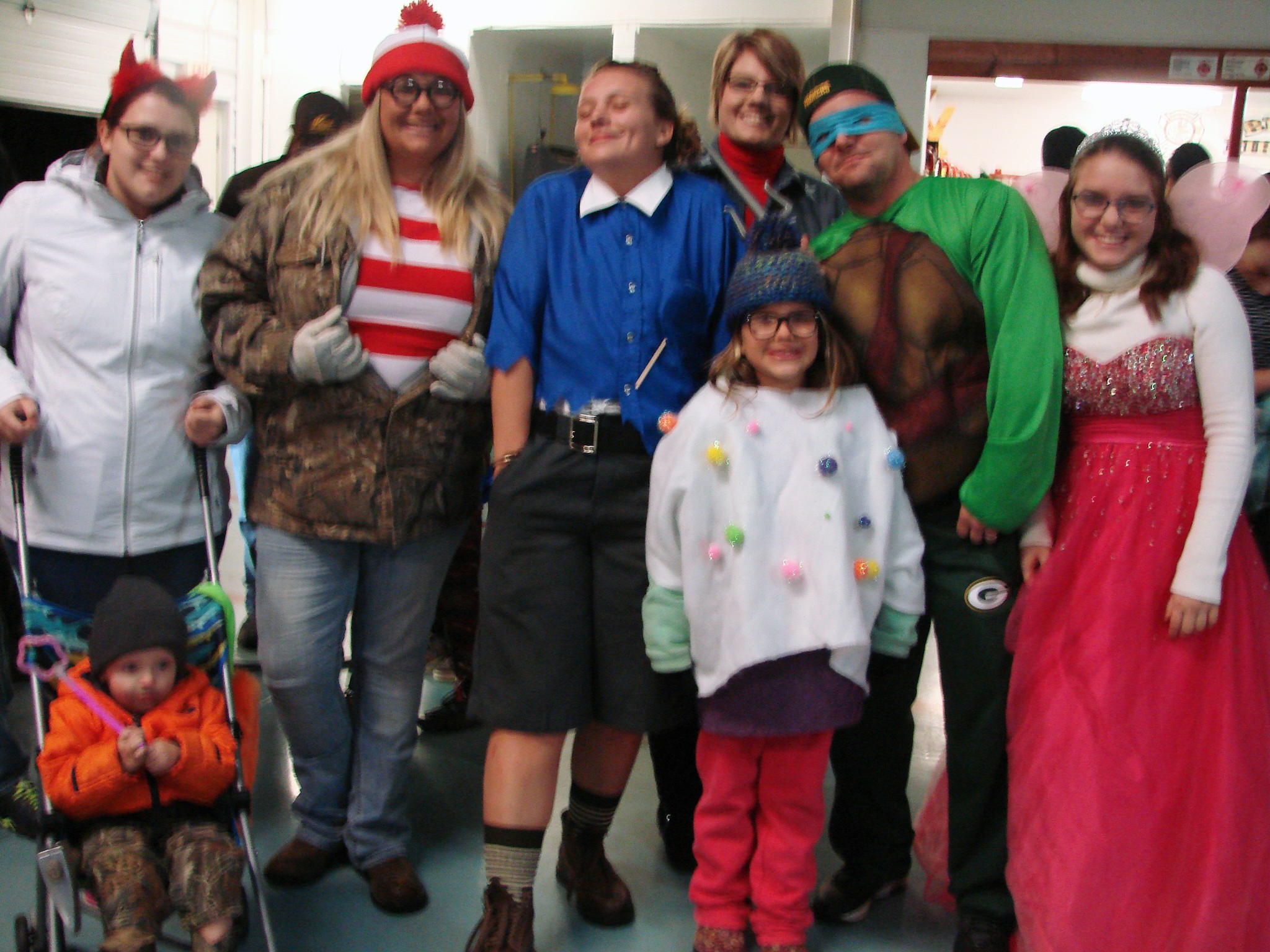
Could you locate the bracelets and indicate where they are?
[490,451,520,468]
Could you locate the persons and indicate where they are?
[641,214,927,951]
[193,5,515,916]
[0,0,1270,952]
[910,125,1270,952]
[462,62,749,952]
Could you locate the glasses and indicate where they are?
[1070,188,1155,224]
[724,76,794,99]
[739,310,820,340]
[380,73,460,110]
[117,126,194,155]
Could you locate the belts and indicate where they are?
[529,409,650,456]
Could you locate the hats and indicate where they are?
[798,65,918,152]
[362,0,474,111]
[294,92,350,145]
[725,218,831,336]
[90,575,187,678]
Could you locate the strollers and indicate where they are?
[4,410,274,952]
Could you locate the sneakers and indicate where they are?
[0,779,46,839]
[815,862,908,922]
[954,920,1009,952]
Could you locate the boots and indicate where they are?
[464,879,536,952]
[556,808,636,927]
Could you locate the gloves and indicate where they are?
[429,332,493,405]
[289,305,370,385]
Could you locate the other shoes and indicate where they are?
[656,797,701,874]
[367,856,427,913]
[694,924,745,952]
[263,839,349,886]
[238,613,259,649]
[425,678,479,734]
